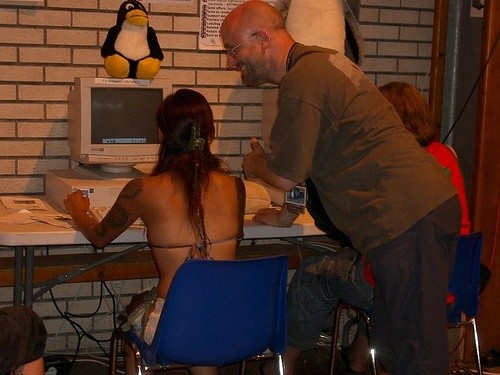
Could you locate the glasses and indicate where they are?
[226,32,271,56]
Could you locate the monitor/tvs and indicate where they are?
[67,76,172,179]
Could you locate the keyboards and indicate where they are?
[89,206,145,229]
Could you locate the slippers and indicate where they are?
[252,361,266,375]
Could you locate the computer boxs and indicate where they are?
[44,168,151,212]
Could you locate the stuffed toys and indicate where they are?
[274,0,364,68]
[100,0,166,80]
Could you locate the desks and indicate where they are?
[0,196,323,312]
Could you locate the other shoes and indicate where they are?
[473,348,500,369]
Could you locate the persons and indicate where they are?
[477,342,500,368]
[217,0,462,375]
[0,304,48,375]
[245,81,472,375]
[64,88,248,375]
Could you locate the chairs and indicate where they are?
[107,253,290,375]
[330,232,485,375]
